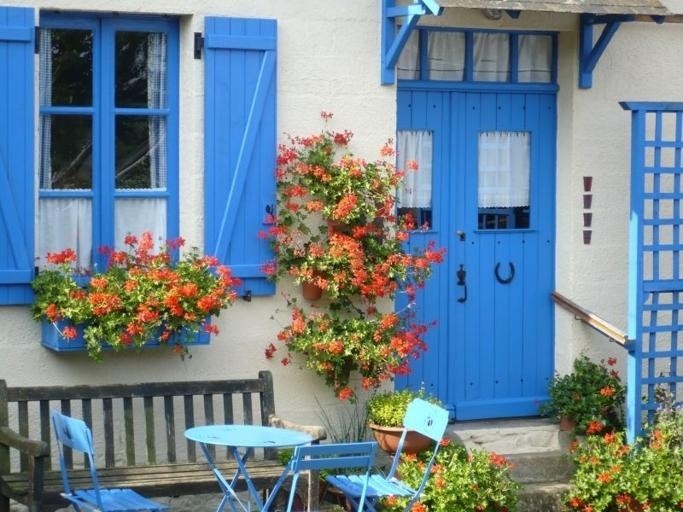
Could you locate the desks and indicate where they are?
[182,422,316,512]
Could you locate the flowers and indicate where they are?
[259,111,447,400]
[29,231,243,364]
[365,379,526,511]
[538,345,683,510]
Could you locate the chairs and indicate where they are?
[52,411,175,511]
[262,399,449,512]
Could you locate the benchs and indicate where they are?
[0,370,326,512]
